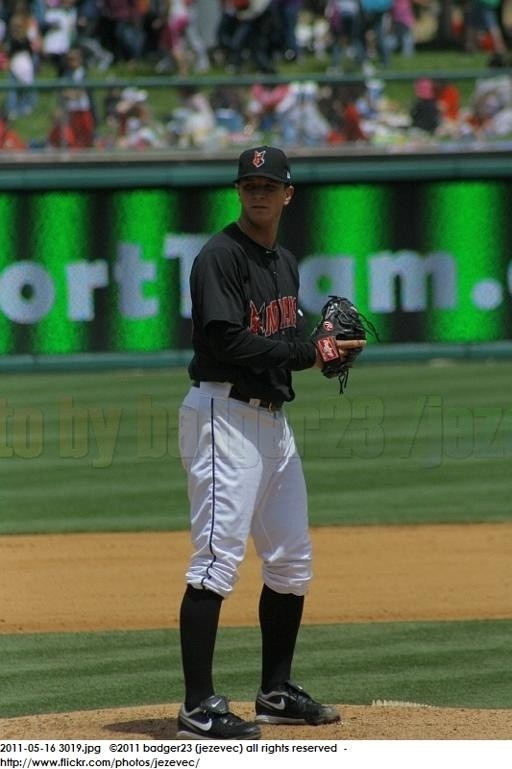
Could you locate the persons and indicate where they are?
[170,143,369,740]
[1,0,512,147]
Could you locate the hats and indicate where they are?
[234,145,293,187]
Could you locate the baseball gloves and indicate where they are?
[311,295,365,378]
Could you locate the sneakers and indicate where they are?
[175,695,260,740]
[254,680,343,726]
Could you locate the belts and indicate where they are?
[191,375,285,413]
